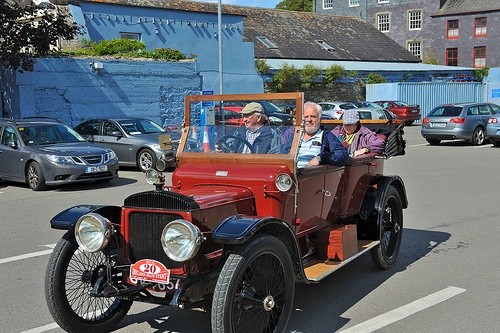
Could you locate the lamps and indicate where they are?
[89,62,104,75]
[156,28,159,35]
[215,32,217,38]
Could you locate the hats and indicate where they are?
[238,102,264,114]
[342,110,359,124]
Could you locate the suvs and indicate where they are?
[259,101,293,125]
[0,116,120,192]
[421,102,500,145]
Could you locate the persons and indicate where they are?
[214,102,281,155]
[281,101,349,170]
[329,108,385,157]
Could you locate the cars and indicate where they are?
[44,92,407,333]
[373,99,421,125]
[214,104,282,126]
[486,111,500,147]
[318,102,359,120]
[72,117,203,172]
[342,102,396,119]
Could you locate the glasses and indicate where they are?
[243,113,253,118]
